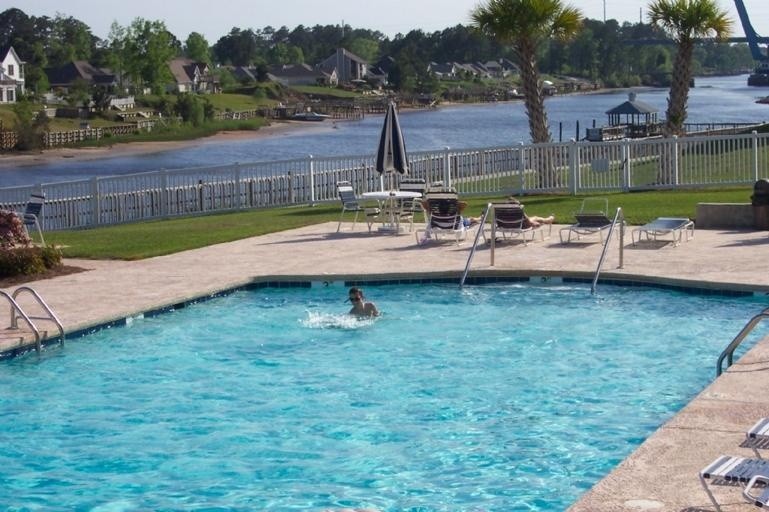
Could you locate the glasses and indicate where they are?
[349,297,360,300]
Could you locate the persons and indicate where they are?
[463,215,485,228]
[507,194,555,228]
[346,287,378,318]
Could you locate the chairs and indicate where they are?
[0,194,46,248]
[700,418,768,512]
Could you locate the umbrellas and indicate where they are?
[374,99,409,191]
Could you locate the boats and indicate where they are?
[747,46,769,87]
[552,93,687,166]
[288,107,332,122]
